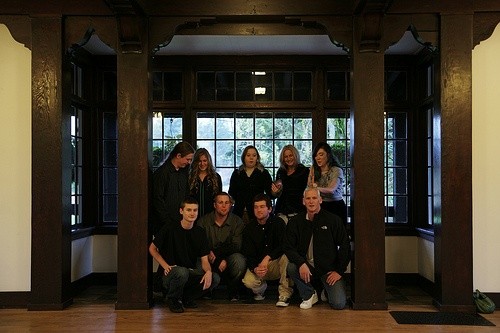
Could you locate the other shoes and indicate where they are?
[276,302,289,306]
[299,290,319,309]
[169,302,199,313]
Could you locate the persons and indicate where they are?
[306,142,348,236]
[150,141,196,298]
[196,191,247,301]
[186,148,222,215]
[228,145,279,225]
[149,197,215,313]
[282,186,352,310]
[276,144,310,223]
[241,193,295,307]
